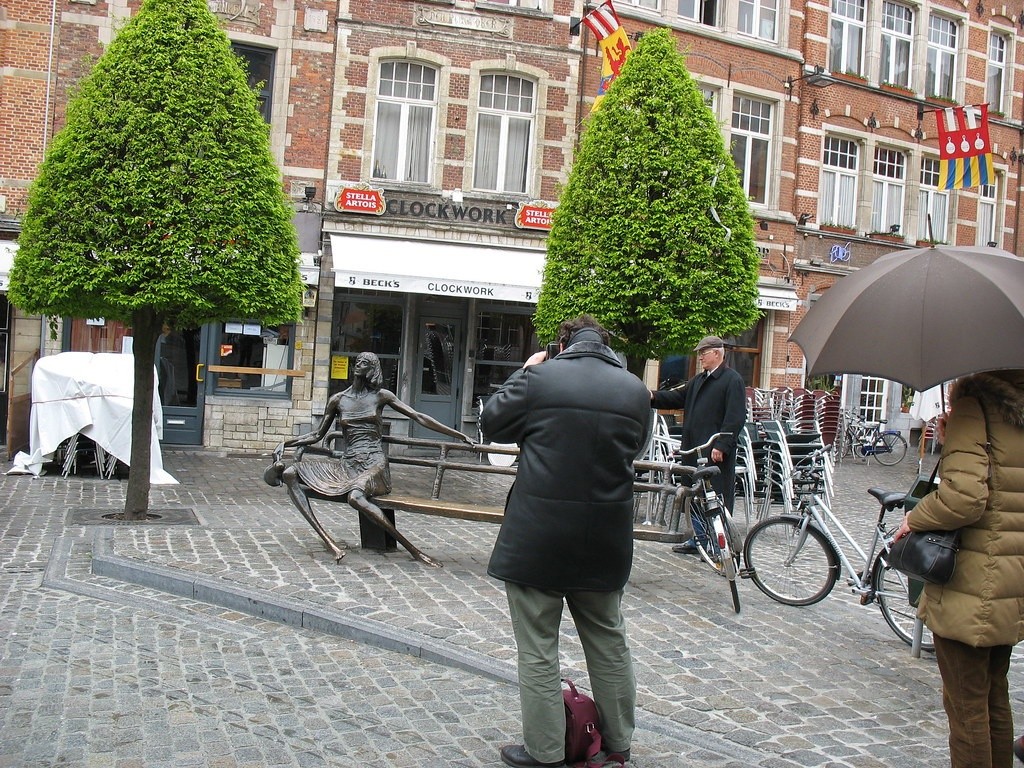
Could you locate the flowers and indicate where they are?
[821,222,858,231]
[845,68,870,81]
[880,79,914,93]
[989,109,1006,117]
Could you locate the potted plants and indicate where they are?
[916,238,947,247]
[926,94,958,108]
[901,385,916,413]
[870,229,905,243]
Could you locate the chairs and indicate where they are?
[630,386,847,539]
[50,432,119,481]
[917,411,949,455]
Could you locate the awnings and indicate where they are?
[329,234,546,304]
[752,286,799,313]
[0,240,20,291]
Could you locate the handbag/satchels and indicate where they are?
[887,528,960,585]
[561,678,625,768]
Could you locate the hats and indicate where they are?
[693,336,724,352]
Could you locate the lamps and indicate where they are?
[799,213,813,226]
[627,31,644,42]
[756,221,768,232]
[987,242,997,247]
[788,65,825,88]
[865,224,901,237]
[305,187,317,203]
[441,189,463,203]
[810,258,823,267]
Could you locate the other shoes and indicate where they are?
[701,550,719,562]
[672,540,699,553]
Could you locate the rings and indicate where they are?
[717,457,720,460]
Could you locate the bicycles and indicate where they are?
[666,432,756,613]
[840,408,908,466]
[743,444,937,654]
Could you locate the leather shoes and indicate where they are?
[501,745,564,768]
[602,743,630,761]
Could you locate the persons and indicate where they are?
[647,336,747,563]
[272,352,480,568]
[894,369,1024,768]
[227,333,254,377]
[154,321,185,405]
[480,313,652,768]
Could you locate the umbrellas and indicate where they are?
[787,243,1024,413]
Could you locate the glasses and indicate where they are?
[698,350,715,356]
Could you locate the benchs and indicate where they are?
[292,430,699,553]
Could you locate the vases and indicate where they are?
[831,71,868,85]
[988,112,1004,120]
[879,85,915,98]
[819,225,856,235]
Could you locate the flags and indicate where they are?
[582,0,633,117]
[935,104,996,190]
